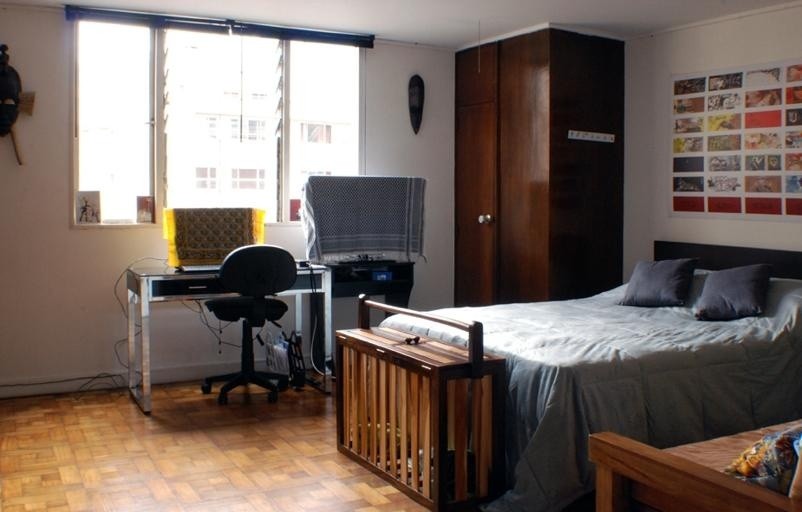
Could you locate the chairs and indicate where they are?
[199,243,298,404]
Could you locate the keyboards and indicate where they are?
[181,266,221,272]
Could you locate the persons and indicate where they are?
[80,195,100,224]
[789,66,802,81]
[676,99,685,115]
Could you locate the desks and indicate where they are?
[121,262,338,416]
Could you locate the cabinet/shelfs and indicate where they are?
[453,20,626,305]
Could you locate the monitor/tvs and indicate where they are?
[172,206,257,265]
[307,175,426,264]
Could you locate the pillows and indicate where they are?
[617,252,774,321]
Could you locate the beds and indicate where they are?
[358,239,801,511]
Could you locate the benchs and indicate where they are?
[583,411,802,512]
